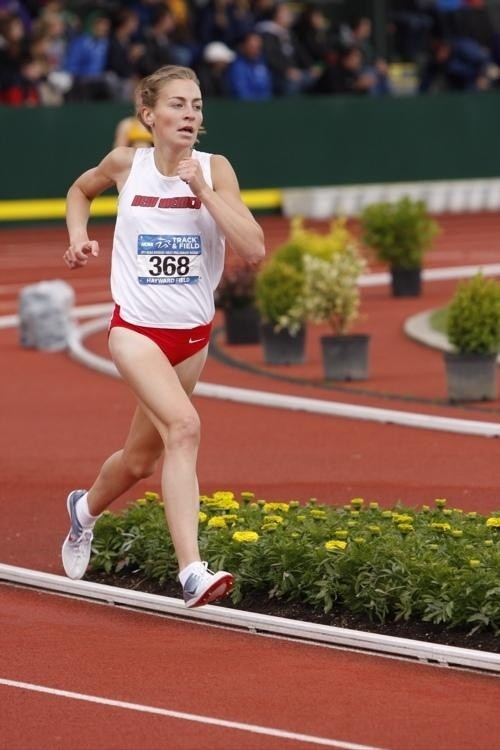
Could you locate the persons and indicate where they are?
[60,66,267,612]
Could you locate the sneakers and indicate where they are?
[182,567,236,610]
[60,488,95,581]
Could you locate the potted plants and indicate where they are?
[363,194,444,299]
[261,216,311,365]
[434,264,500,403]
[279,252,375,382]
[215,252,263,344]
